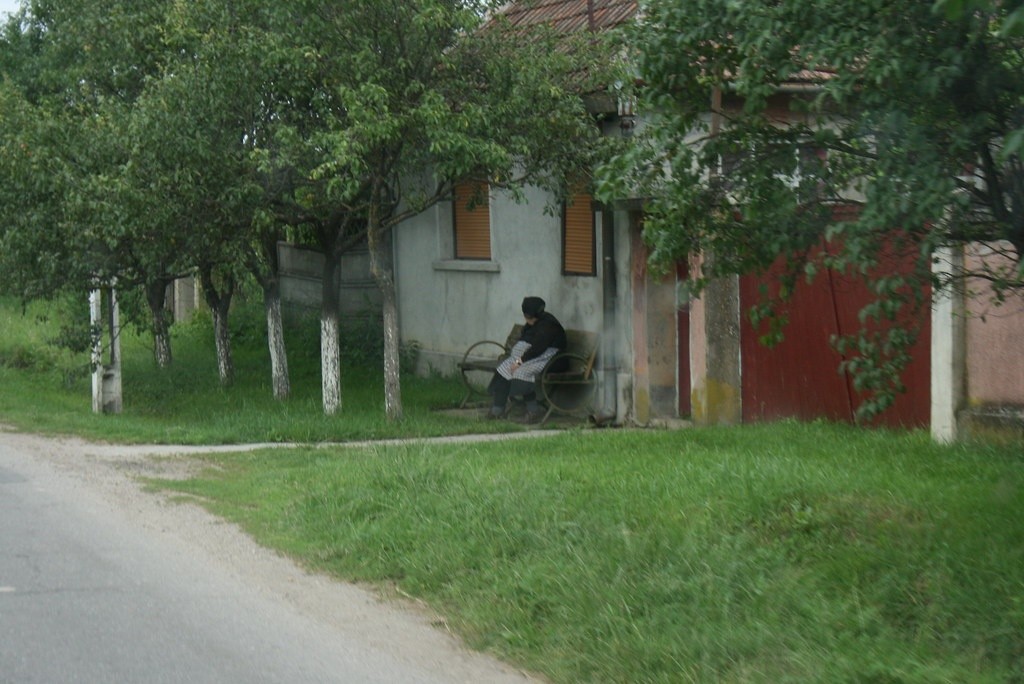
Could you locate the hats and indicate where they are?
[522,297,545,316]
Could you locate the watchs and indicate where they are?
[515,359,522,366]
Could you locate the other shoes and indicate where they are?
[513,412,540,423]
[488,409,506,420]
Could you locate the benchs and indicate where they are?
[455,325,601,428]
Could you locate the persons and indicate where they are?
[485,296,568,424]
